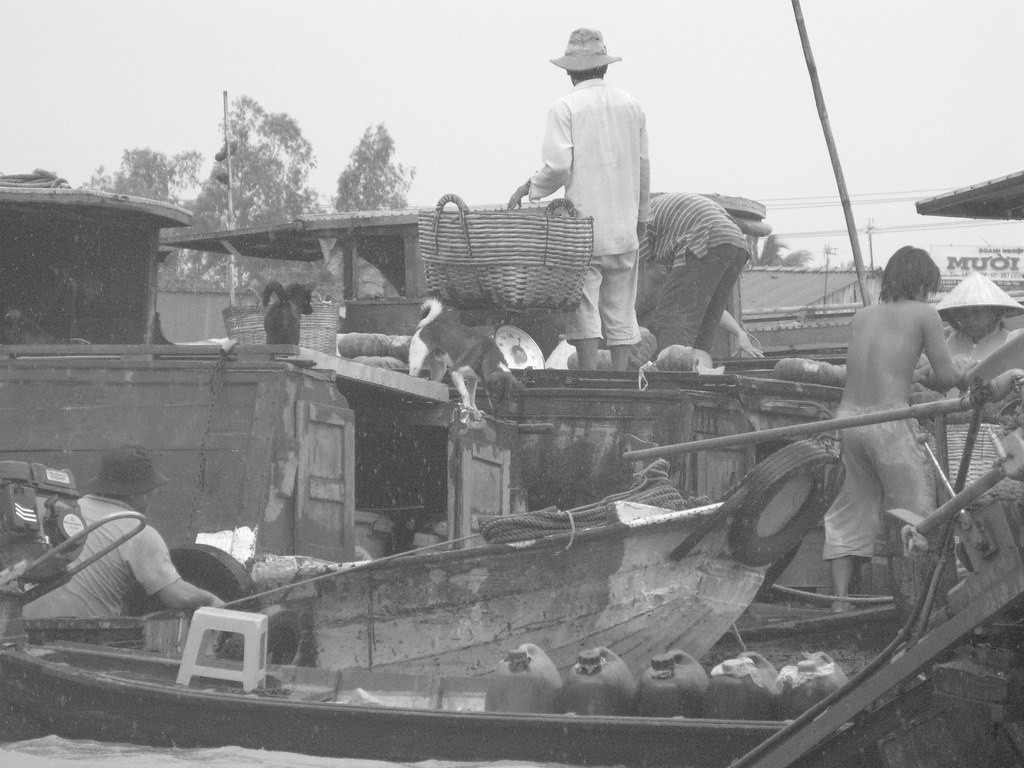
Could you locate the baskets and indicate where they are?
[416,192,596,311]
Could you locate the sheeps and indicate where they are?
[262,281,313,345]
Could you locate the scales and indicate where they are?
[453,305,545,372]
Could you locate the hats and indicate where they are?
[936,271,1024,311]
[87,444,172,496]
[549,28,622,71]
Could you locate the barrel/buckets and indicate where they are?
[632,649,708,719]
[558,646,635,716]
[700,651,779,721]
[483,643,562,714]
[774,652,849,722]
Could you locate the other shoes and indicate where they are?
[831,601,851,612]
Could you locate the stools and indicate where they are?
[175,606,268,693]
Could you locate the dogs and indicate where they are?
[408,297,526,421]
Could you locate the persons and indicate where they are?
[21,447,226,619]
[638,191,750,356]
[504,28,652,371]
[823,246,971,613]
[637,258,765,358]
[925,268,1024,399]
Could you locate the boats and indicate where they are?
[1,165,1024,763]
